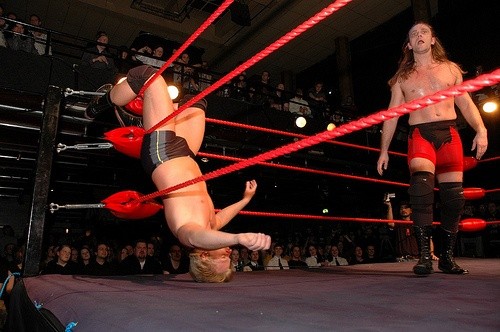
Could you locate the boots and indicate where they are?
[438,225,469,274]
[412,224,433,274]
[115,105,144,128]
[84,83,116,120]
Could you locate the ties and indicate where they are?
[335,257,340,266]
[256,264,258,268]
[315,257,320,263]
[279,258,283,269]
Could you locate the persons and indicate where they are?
[0,192,500,332]
[0,8,54,93]
[77,31,381,133]
[84,65,271,283]
[377,21,488,274]
